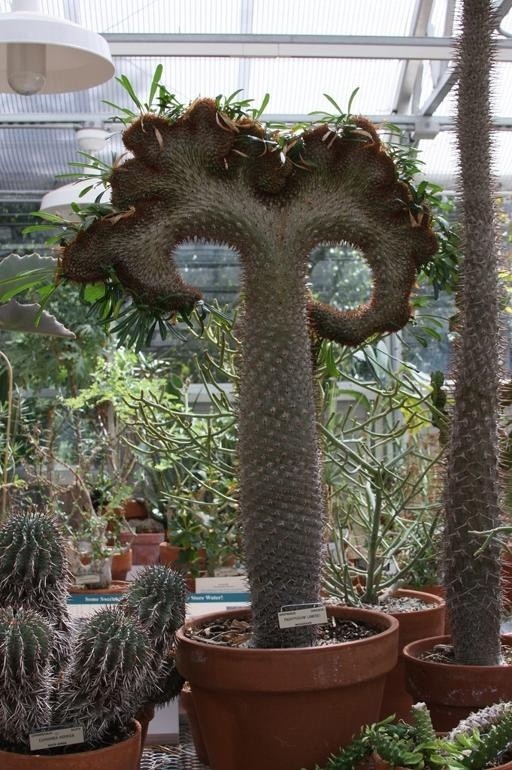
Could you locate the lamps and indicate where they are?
[39,128,121,227]
[3,1,118,97]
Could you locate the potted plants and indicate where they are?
[327,698,511,770]
[4,505,188,770]
[403,2,510,737]
[2,64,469,769]
[321,453,447,730]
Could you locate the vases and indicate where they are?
[84,529,238,593]
